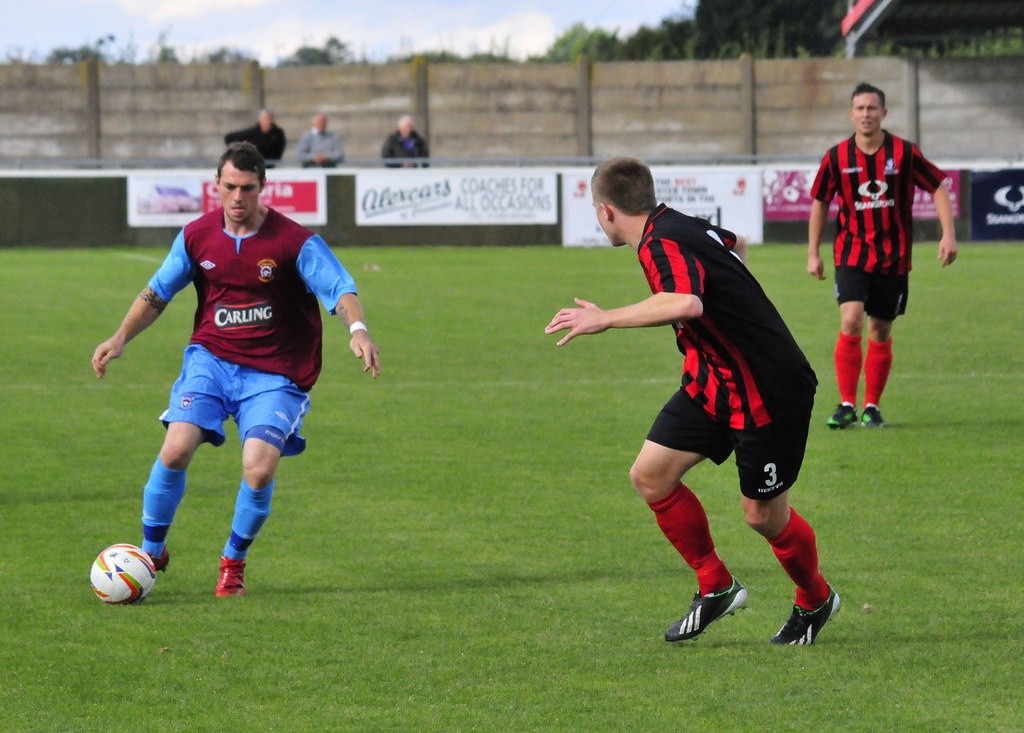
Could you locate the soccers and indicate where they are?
[90,542,157,605]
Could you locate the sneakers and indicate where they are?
[826,403,858,430]
[146,546,170,572]
[214,554,246,598]
[769,582,842,645]
[860,406,889,429]
[664,574,748,641]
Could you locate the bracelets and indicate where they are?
[349,321,367,335]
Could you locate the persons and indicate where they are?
[382,115,430,168]
[807,82,957,428]
[92,141,380,597]
[545,157,839,646]
[223,110,286,168]
[297,112,344,168]
